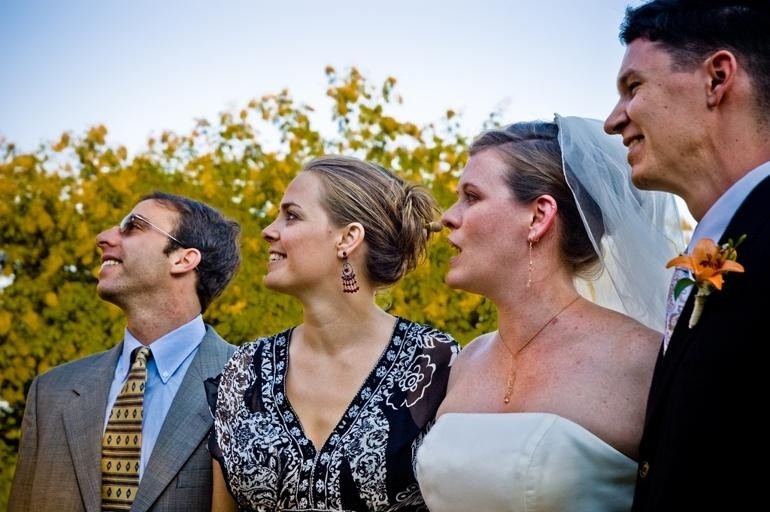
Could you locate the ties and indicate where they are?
[664,248,696,355]
[99,345,150,510]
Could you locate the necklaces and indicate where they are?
[496,289,585,404]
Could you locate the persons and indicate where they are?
[603,0,770,512]
[6,193,248,511]
[415,114,667,512]
[206,151,459,511]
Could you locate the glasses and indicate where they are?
[118,212,186,245]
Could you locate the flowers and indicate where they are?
[665,232,746,332]
